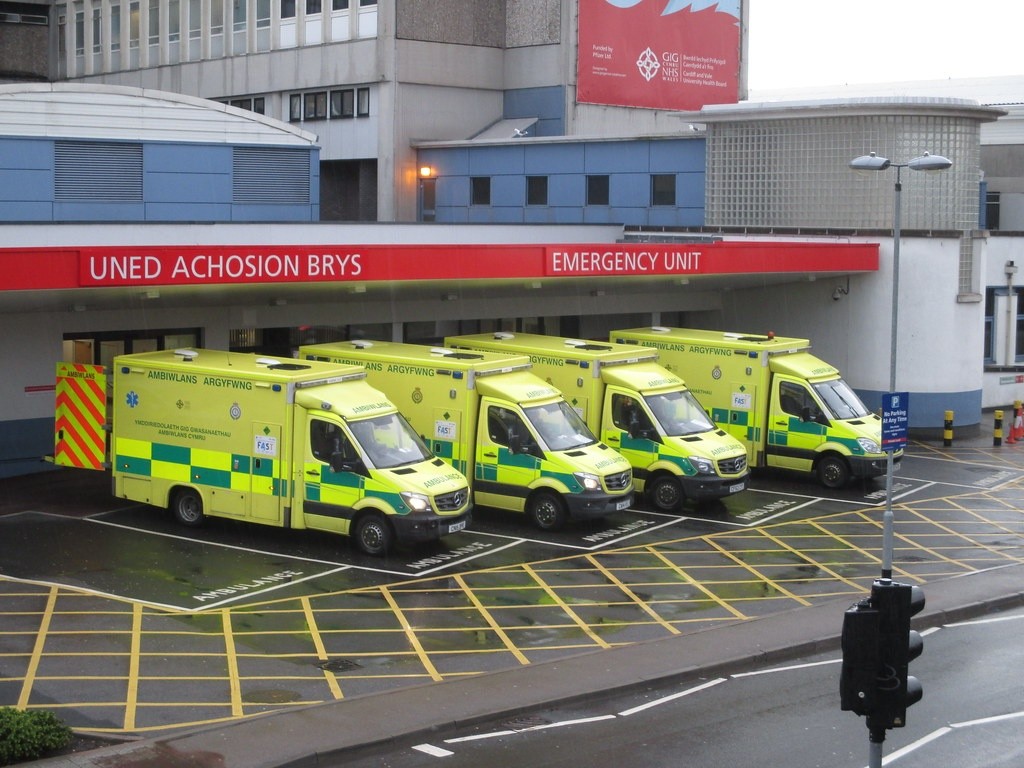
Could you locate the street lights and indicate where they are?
[838,144,953,768]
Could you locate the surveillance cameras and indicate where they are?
[832,292,841,300]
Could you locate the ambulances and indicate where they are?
[35,347,472,561]
[443,330,752,514]
[607,323,905,495]
[292,340,636,534]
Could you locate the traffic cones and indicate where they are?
[1004,423,1018,445]
[1013,407,1024,441]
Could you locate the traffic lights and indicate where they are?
[868,576,928,728]
